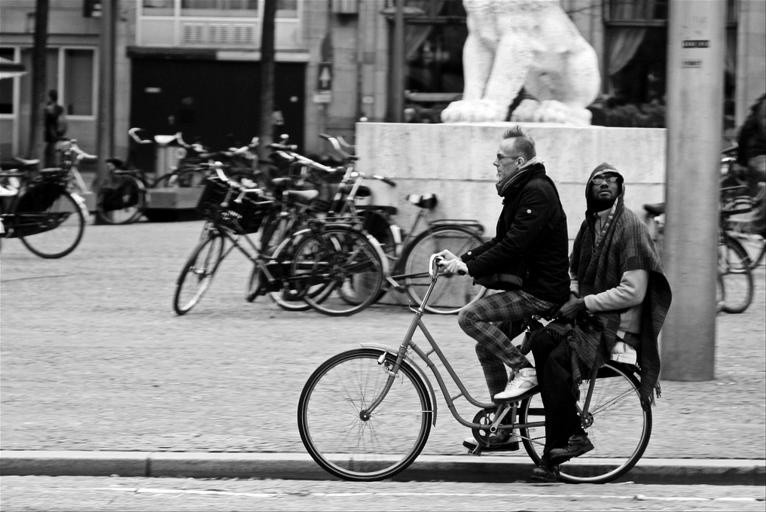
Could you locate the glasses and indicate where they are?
[592,176,618,185]
[497,154,517,161]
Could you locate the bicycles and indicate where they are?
[644,145,766,317]
[297,253,652,483]
[0,157,88,259]
[62,139,146,225]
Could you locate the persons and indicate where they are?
[530,161,672,479]
[41,88,69,172]
[430,125,571,451]
[733,92,766,207]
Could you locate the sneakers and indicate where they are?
[493,367,541,401]
[530,459,561,482]
[463,432,519,451]
[549,432,595,463]
